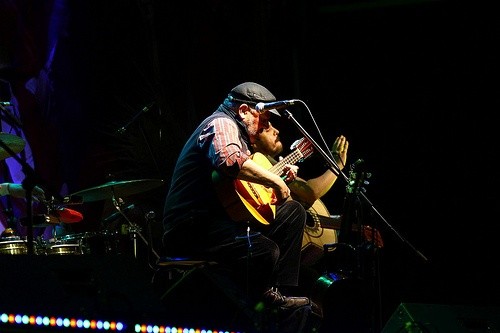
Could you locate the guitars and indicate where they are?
[211,138,313,230]
[298,199,384,267]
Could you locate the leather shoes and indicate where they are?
[260,286,310,316]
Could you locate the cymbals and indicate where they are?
[70,179,166,203]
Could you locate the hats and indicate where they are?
[231,82,282,117]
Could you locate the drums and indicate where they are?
[0,234,93,256]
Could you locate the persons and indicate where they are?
[248,120,349,281]
[161,82,324,333]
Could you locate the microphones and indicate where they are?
[255,100,293,111]
[103,204,135,223]
[116,108,150,135]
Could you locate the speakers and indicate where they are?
[379,302,500,333]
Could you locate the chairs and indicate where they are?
[112,194,218,323]
